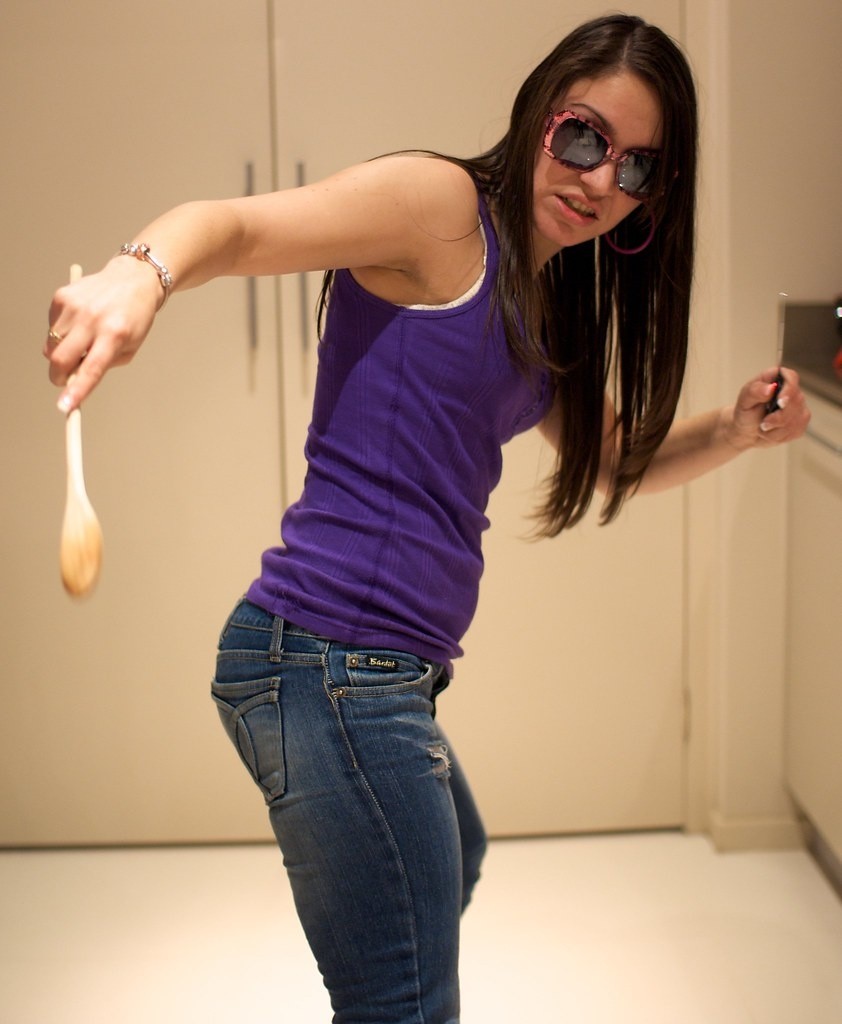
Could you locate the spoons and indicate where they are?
[59,264,102,599]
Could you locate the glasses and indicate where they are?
[542,110,679,202]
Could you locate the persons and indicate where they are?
[40,11,813,1024]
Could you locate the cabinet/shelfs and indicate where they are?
[777,420,842,893]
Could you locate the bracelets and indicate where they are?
[112,242,171,305]
